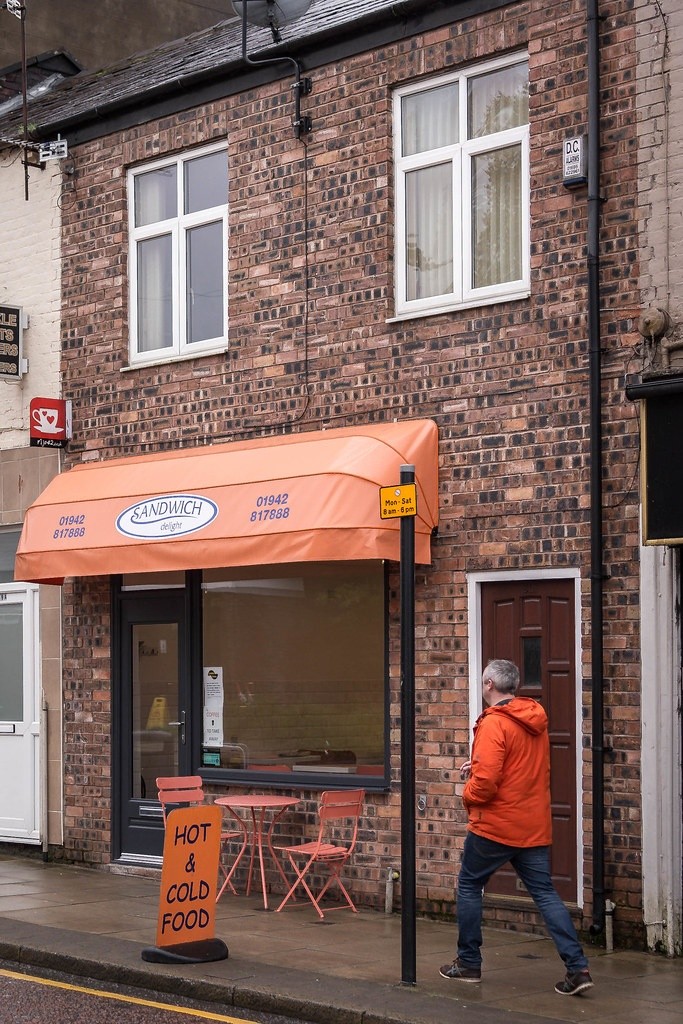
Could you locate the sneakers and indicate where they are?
[555,967,594,995]
[439,961,483,983]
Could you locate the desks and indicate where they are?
[210,793,302,911]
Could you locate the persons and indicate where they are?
[438,659,595,995]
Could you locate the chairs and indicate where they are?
[274,788,368,918]
[156,776,242,896]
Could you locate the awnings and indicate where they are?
[14,419,439,587]
